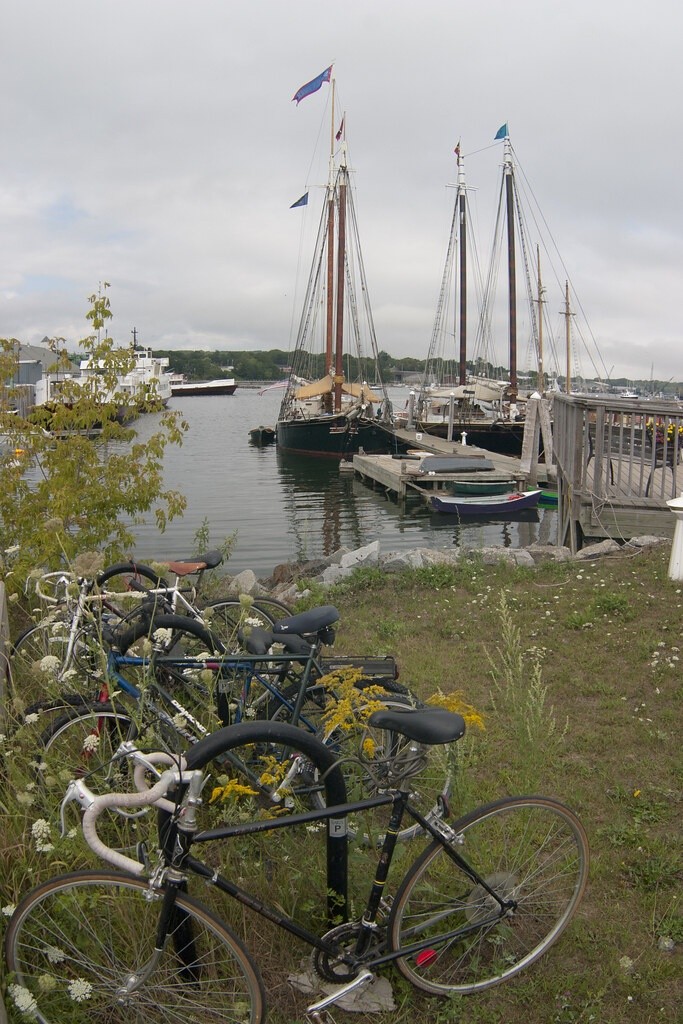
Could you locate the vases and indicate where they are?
[655,440,680,460]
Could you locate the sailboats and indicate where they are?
[277,75,395,471]
[397,119,650,451]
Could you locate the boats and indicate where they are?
[451,481,518,496]
[431,490,542,513]
[527,485,558,501]
[170,374,237,396]
[248,426,276,447]
[367,384,382,389]
[619,392,638,399]
[42,326,173,426]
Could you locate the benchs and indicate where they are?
[584,420,680,498]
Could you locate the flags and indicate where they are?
[493,124,509,140]
[335,118,343,141]
[453,142,460,167]
[291,66,332,106]
[290,192,308,208]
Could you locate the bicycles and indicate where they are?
[4,549,589,1024]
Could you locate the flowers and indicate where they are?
[642,420,683,443]
